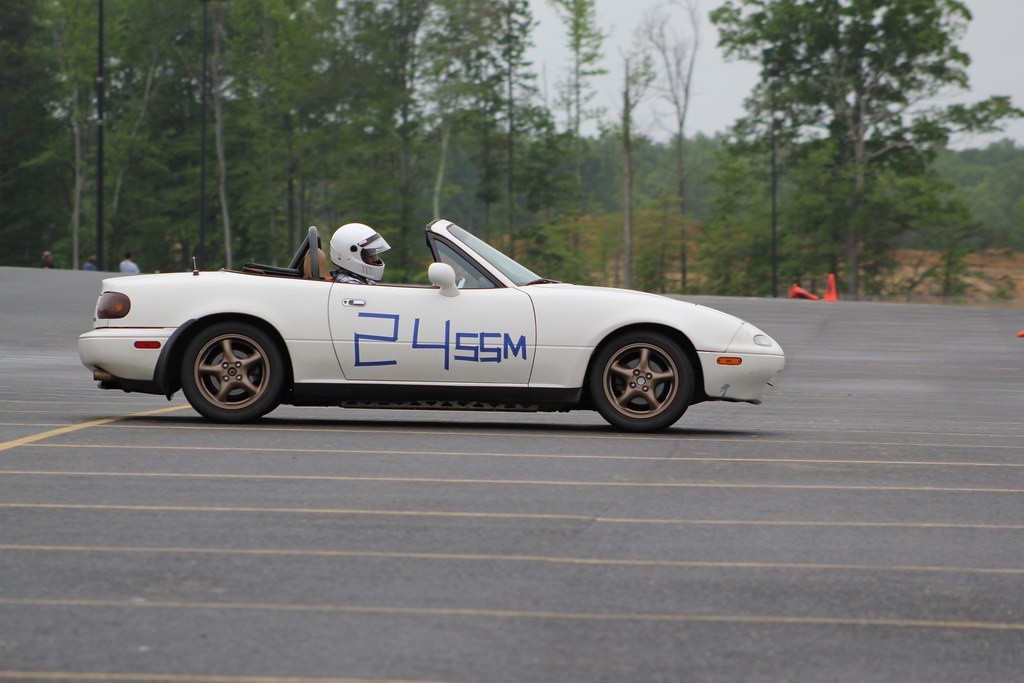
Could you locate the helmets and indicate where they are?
[330,222,391,281]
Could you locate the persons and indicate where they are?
[329,223,468,284]
[40,252,163,275]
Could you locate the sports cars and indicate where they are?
[75,217,789,430]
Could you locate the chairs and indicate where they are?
[305,224,331,281]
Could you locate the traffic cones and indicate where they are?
[789,283,819,302]
[822,273,838,301]
[1017,330,1024,338]
